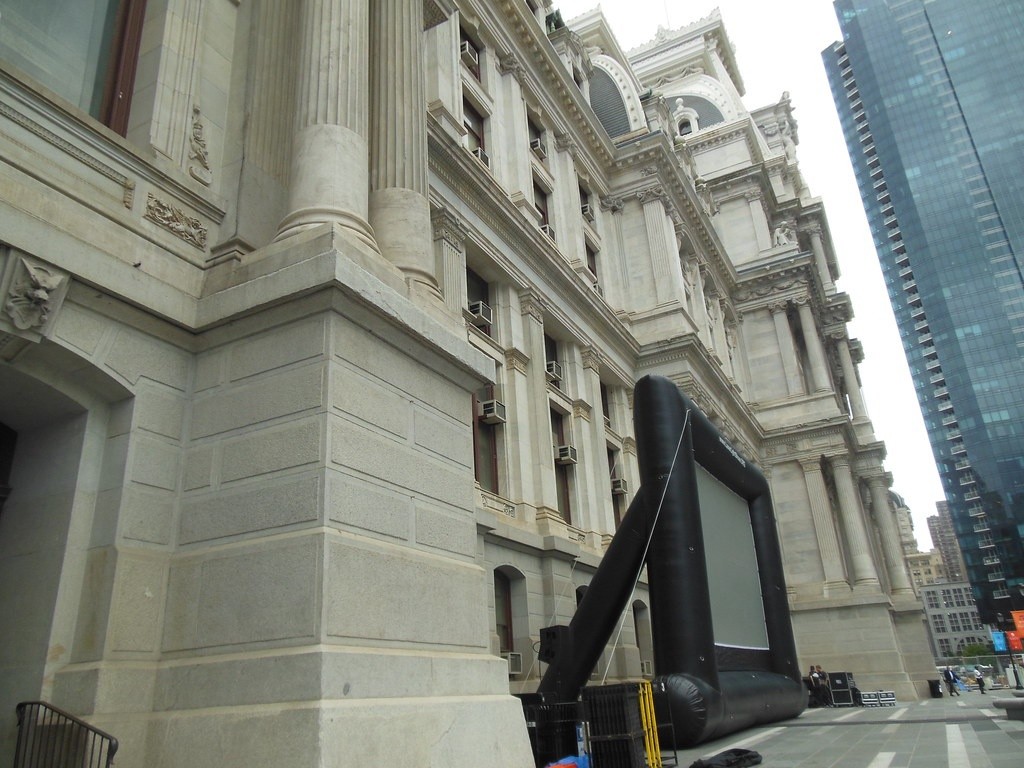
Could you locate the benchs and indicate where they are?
[993,697,1024,720]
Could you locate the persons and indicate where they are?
[815,665,836,708]
[974,666,987,694]
[944,666,960,696]
[808,665,834,708]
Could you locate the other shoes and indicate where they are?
[981,691,985,694]
[950,694,953,696]
[957,694,960,696]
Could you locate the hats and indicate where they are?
[975,665,979,668]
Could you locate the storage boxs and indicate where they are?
[855,692,879,704]
[878,691,896,703]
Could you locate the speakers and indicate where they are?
[538,626,572,668]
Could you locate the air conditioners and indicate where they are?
[592,661,600,676]
[470,300,493,326]
[582,204,595,221]
[501,652,522,674]
[547,361,563,381]
[530,138,547,159]
[557,445,578,464]
[611,477,629,494]
[594,284,604,298]
[461,41,479,67]
[472,147,491,171]
[540,224,556,244]
[641,660,652,676]
[478,399,507,424]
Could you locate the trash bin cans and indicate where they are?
[927,680,942,698]
[529,702,579,768]
[580,682,648,768]
